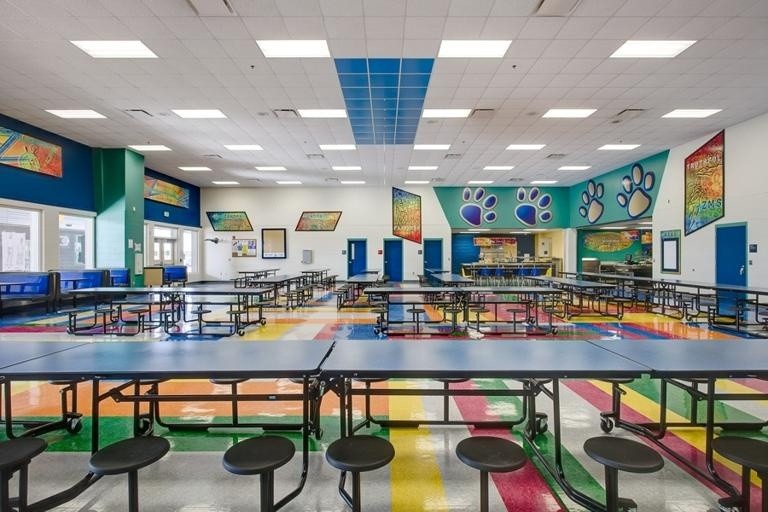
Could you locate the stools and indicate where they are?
[1,268,767,512]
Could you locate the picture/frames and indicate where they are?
[391,186,422,245]
[0,124,64,179]
[144,174,191,209]
[261,227,287,258]
[294,211,343,232]
[205,211,253,231]
[683,130,726,237]
